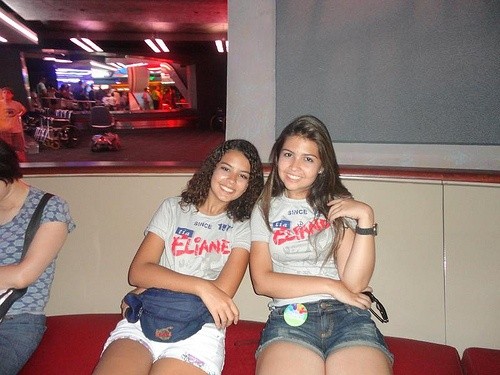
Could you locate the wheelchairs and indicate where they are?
[91,106,121,152]
[34,108,81,149]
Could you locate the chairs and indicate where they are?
[90,105,115,152]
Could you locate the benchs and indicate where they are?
[0,167,500,375]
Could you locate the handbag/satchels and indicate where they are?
[124,287,214,343]
[0,287,28,323]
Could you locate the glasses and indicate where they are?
[363,292,389,323]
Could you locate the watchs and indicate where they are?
[355,223,378,236]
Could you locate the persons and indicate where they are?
[93,137,264,374]
[143,83,181,110]
[31,78,129,110]
[249,115,395,375]
[0,88,27,163]
[0,139,77,374]
